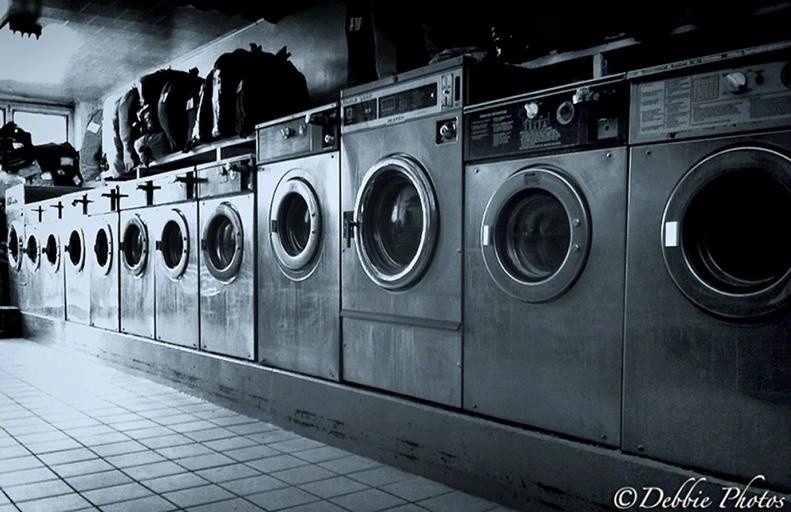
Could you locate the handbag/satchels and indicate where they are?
[78,43,310,184]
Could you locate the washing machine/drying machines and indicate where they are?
[5,44,790,489]
